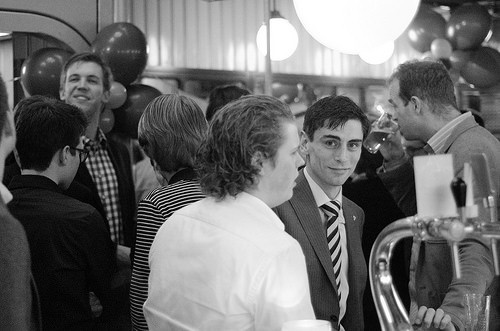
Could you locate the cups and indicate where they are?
[364,112,399,153]
[464,294,491,331]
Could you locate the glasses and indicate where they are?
[71,145,90,162]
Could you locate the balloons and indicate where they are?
[21,23,164,137]
[407,0,500,91]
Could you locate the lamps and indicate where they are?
[256,0,298,99]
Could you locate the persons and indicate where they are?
[376,59,500,331]
[58,51,137,331]
[0,75,35,331]
[3,95,102,211]
[131,140,162,200]
[142,93,316,331]
[4,94,121,331]
[205,83,252,123]
[341,119,414,331]
[271,96,368,331]
[129,93,211,331]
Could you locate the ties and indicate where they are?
[321,200,344,304]
